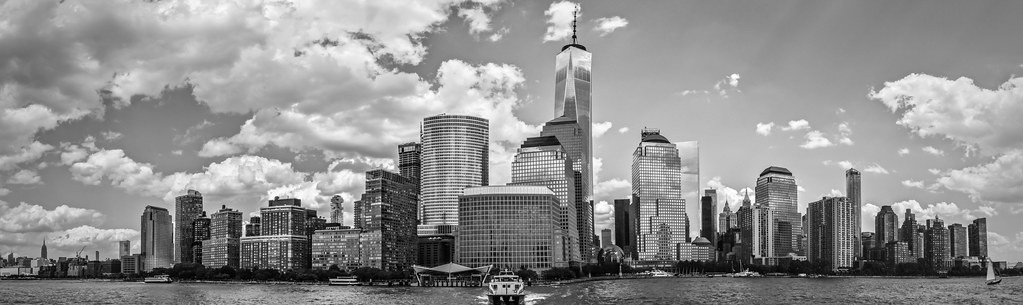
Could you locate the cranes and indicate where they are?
[76,245,86,259]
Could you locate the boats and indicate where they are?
[328,275,365,286]
[648,268,674,278]
[734,267,761,279]
[487,268,526,305]
[144,273,173,283]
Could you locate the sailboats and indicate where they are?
[985,257,1003,286]
